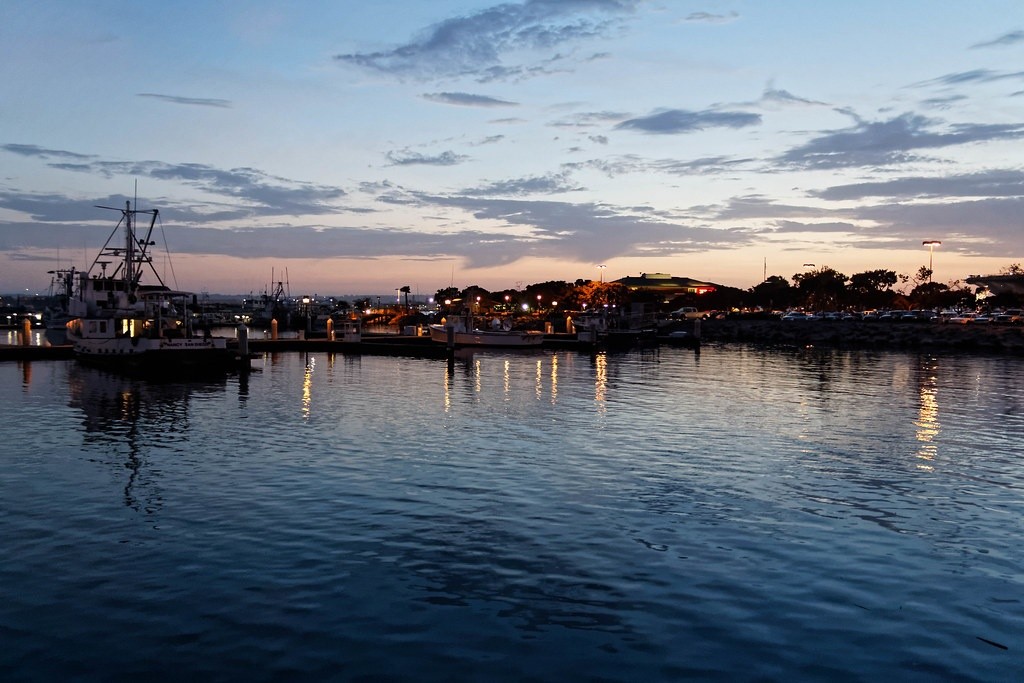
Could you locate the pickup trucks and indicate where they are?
[671,307,706,321]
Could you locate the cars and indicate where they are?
[783,308,1024,324]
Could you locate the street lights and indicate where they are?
[537,295,541,317]
[302,295,309,340]
[477,297,481,315]
[552,301,557,335]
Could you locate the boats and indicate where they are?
[66,290,226,357]
[571,315,658,341]
[429,308,545,345]
[44,180,179,329]
